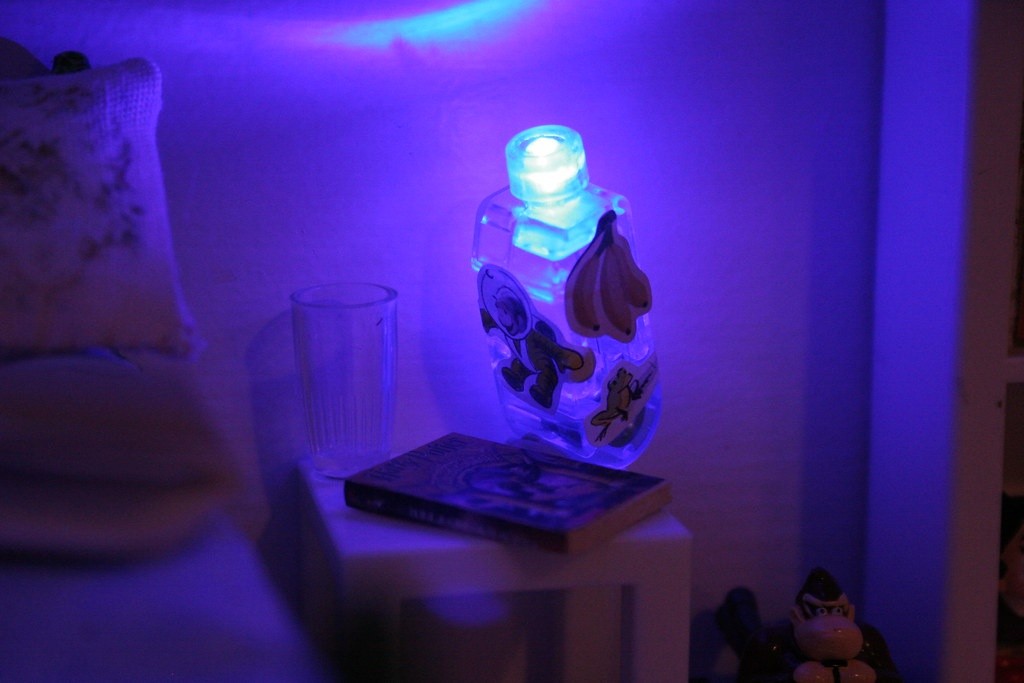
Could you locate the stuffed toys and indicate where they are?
[784,566,878,683]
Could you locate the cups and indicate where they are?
[287,282,400,474]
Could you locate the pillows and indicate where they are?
[0,57,186,357]
[0,147,255,485]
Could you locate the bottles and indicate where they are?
[470,126,660,469]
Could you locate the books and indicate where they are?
[344,431,673,554]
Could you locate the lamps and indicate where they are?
[468,126,664,470]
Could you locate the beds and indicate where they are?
[0,33,332,682]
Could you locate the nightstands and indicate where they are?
[293,446,693,682]
